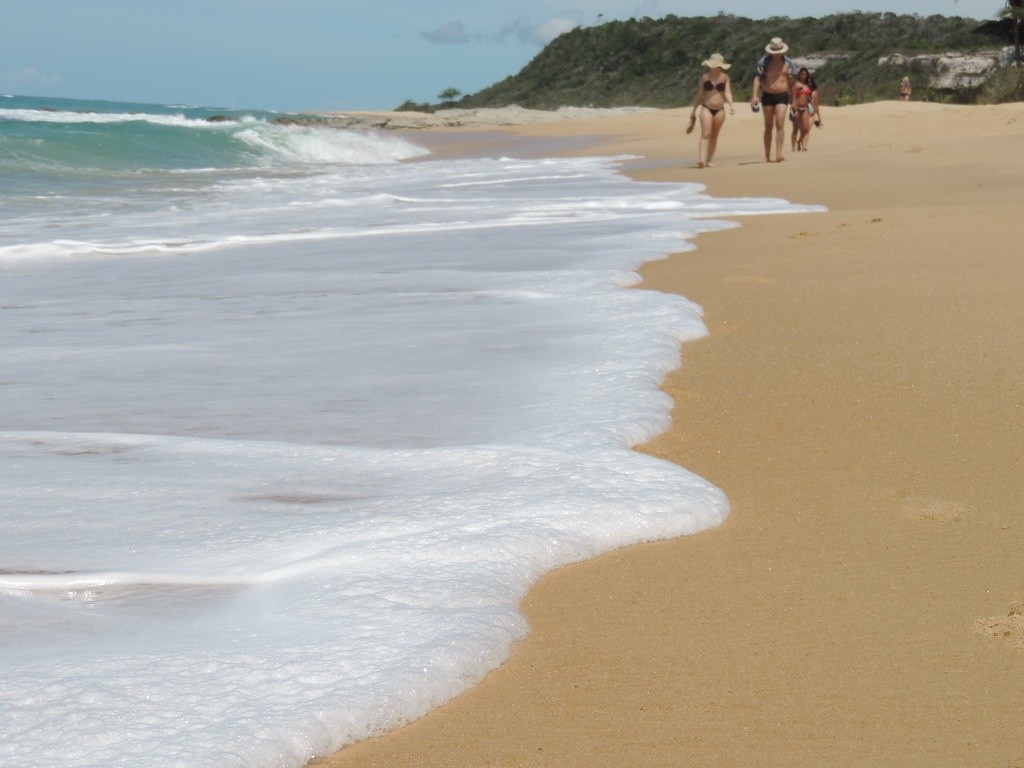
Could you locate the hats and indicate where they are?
[701,54,730,70]
[765,38,788,54]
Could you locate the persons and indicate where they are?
[690,53,735,168]
[751,37,800,163]
[791,66,821,153]
[900,77,911,101]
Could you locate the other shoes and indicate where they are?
[687,117,697,134]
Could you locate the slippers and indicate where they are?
[789,108,796,121]
[751,103,759,112]
